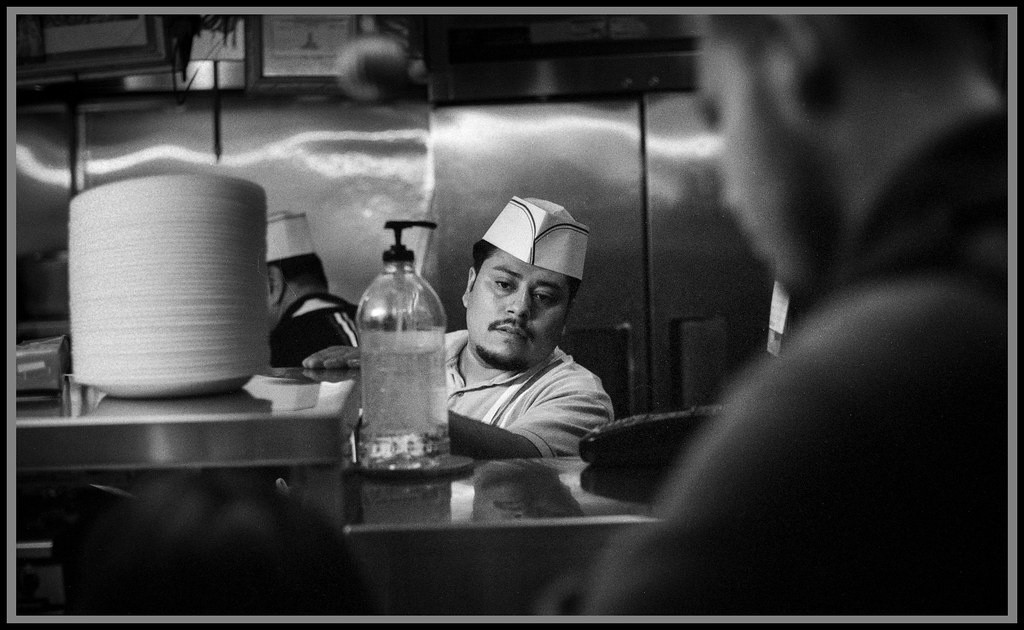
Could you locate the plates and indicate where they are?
[69,173,266,399]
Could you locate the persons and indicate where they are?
[266,211,359,367]
[538,14,1008,616]
[301,194,615,458]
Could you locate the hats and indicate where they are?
[481,195,590,282]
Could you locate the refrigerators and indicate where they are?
[421,90,762,450]
